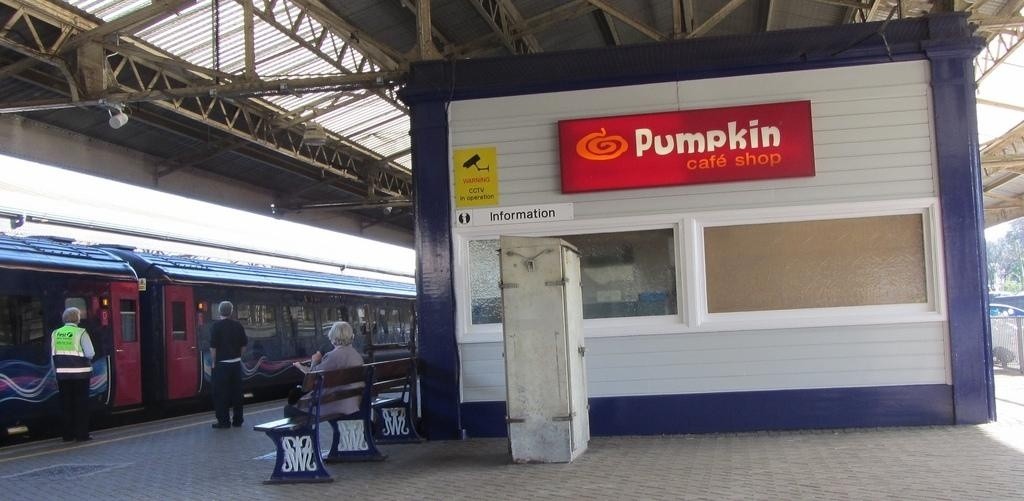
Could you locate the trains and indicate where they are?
[0,231,422,445]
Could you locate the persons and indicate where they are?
[208,299,247,430]
[282,321,364,418]
[48,306,95,442]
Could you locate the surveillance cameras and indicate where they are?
[104,103,131,130]
[382,206,394,215]
[463,153,489,172]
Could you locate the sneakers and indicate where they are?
[70,434,95,442]
[212,421,231,428]
[232,419,247,426]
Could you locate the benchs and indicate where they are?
[253,356,425,484]
